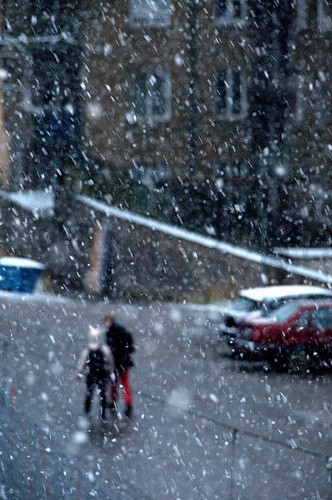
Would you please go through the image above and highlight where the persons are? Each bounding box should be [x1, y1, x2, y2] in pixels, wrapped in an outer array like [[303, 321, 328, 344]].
[[76, 326, 116, 417], [101, 314, 134, 416]]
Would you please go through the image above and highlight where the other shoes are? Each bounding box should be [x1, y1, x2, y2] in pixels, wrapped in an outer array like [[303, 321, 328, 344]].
[[84, 405, 91, 411], [124, 403, 133, 415], [107, 401, 115, 409], [101, 410, 107, 418]]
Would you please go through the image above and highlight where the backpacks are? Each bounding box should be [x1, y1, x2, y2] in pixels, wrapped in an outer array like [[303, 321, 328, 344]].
[[86, 343, 104, 383]]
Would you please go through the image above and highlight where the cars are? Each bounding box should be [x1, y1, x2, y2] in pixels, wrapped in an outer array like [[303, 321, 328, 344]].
[[238, 298, 332, 374]]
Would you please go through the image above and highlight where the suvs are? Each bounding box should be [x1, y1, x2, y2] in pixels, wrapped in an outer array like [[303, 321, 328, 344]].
[[220, 283, 331, 354]]
